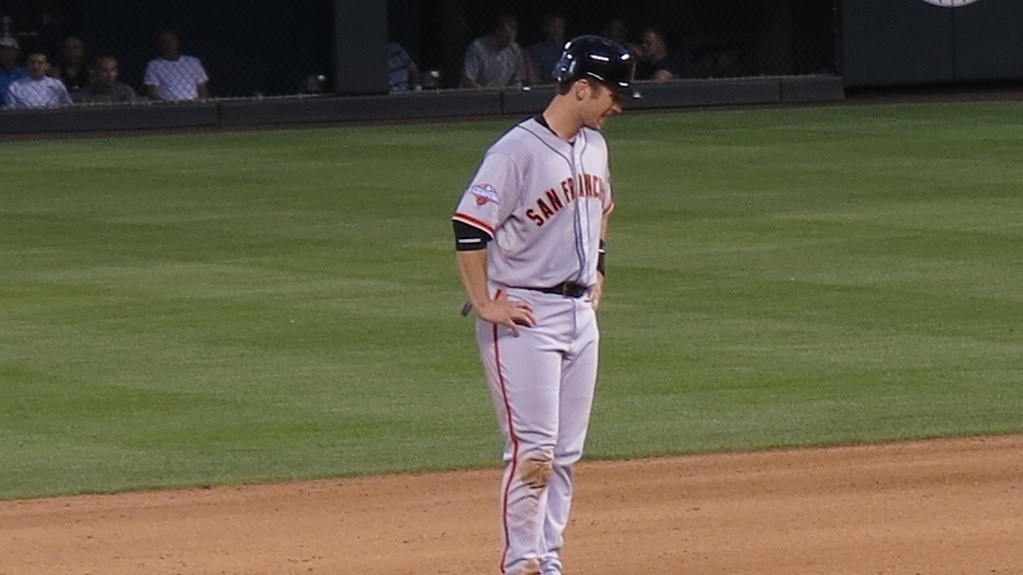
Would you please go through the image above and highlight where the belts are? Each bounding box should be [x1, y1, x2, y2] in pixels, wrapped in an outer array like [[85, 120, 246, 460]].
[[526, 272, 587, 298]]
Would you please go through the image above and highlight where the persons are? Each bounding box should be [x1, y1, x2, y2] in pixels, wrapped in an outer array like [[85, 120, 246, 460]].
[[452, 37, 642, 575], [456, 12, 676, 90], [0, 25, 210, 110]]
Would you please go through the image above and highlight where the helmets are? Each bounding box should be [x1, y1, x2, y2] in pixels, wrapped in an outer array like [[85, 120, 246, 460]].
[[553, 36, 642, 99]]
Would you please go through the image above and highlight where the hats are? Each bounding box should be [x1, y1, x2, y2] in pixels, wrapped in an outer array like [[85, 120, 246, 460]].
[[0, 36, 19, 49]]
